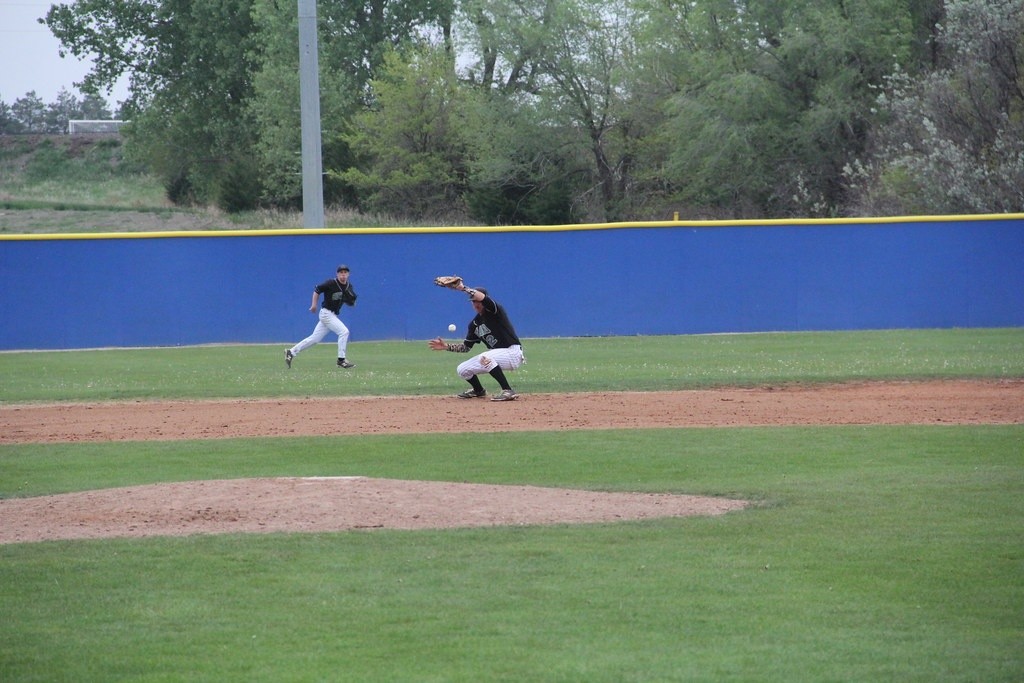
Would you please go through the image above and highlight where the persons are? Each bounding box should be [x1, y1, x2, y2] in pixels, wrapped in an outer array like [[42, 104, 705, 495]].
[[285, 265, 357, 368], [429, 277, 525, 400]]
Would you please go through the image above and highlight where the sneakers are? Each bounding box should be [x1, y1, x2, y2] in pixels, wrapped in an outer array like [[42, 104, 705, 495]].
[[283, 348, 294, 369], [457, 387, 487, 399], [490, 389, 520, 401], [337, 359, 356, 369]]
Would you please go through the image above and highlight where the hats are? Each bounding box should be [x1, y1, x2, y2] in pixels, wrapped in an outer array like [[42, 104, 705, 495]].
[[467, 286, 488, 302], [336, 265, 350, 273]]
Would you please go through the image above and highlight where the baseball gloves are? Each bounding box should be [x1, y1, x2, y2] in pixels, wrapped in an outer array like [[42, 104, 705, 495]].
[[345, 289, 359, 306], [433, 273, 463, 290]]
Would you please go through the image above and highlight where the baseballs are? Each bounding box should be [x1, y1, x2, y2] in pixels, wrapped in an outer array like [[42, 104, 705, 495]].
[[448, 324, 456, 332]]
